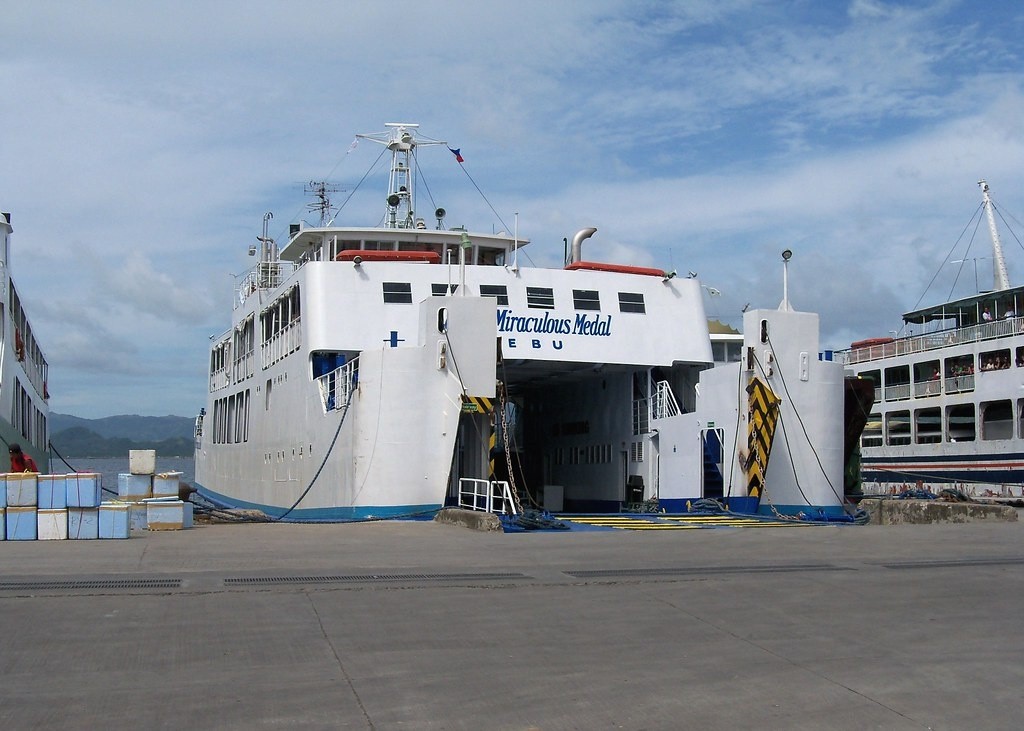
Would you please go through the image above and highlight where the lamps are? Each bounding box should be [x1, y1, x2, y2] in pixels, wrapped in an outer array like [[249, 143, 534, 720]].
[[687, 268, 698, 279], [353, 256, 362, 268], [208, 334, 214, 342], [782, 249, 793, 260], [461, 232, 473, 250], [387, 194, 400, 207]]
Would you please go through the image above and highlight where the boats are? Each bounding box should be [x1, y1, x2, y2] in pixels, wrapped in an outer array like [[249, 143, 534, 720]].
[[190, 122, 876, 533], [709, 180, 1024, 504], [0, 212, 51, 478]]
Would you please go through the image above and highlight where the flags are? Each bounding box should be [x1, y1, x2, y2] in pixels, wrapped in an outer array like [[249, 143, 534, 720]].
[[456, 154, 464, 163], [449, 147, 460, 155]]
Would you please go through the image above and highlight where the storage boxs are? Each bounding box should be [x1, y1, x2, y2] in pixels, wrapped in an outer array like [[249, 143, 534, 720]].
[[38, 509, 68, 540], [152, 475, 178, 498], [37, 475, 66, 508], [102, 502, 147, 529], [66, 473, 103, 508], [118, 474, 151, 502], [6, 506, 37, 540], [147, 499, 183, 531], [129, 449, 155, 474], [0, 508, 6, 541], [99, 505, 132, 539], [69, 507, 99, 539], [0, 471, 7, 508], [7, 472, 42, 506]]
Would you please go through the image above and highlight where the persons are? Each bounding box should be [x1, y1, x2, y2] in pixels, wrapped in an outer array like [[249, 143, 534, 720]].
[[7, 443, 39, 472], [983, 307, 993, 320], [950, 354, 1024, 377], [932, 367, 940, 379], [1000, 306, 1014, 319]]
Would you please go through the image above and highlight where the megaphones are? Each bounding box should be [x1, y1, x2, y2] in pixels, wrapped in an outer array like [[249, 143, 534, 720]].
[[436, 208, 446, 219]]
